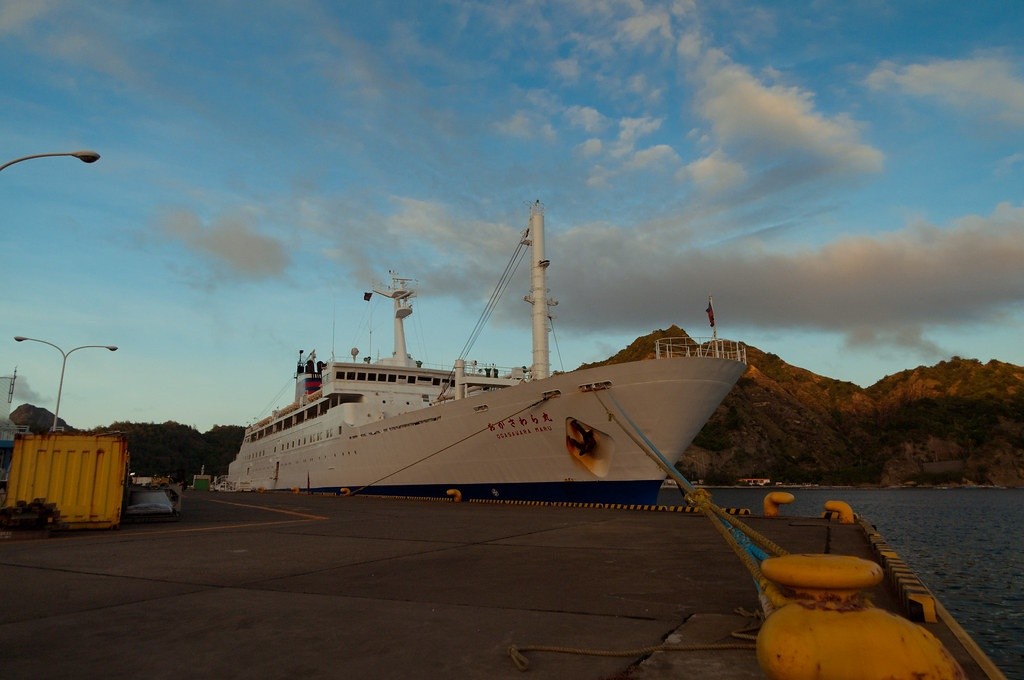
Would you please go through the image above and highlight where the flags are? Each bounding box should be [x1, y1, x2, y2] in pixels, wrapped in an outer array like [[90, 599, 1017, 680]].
[[706, 302, 715, 328], [364, 292, 373, 301]]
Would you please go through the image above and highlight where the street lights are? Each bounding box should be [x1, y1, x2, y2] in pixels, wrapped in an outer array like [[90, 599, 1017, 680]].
[[15, 336, 119, 433]]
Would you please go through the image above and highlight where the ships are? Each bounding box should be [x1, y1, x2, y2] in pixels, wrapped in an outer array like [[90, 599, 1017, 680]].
[[210, 198, 750, 511]]
